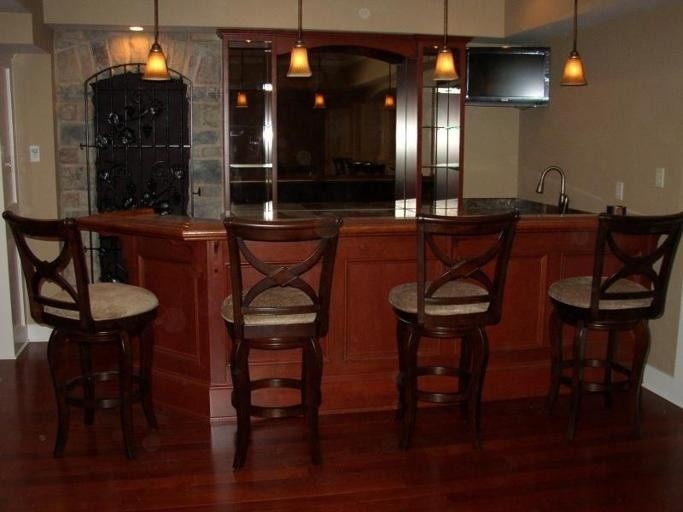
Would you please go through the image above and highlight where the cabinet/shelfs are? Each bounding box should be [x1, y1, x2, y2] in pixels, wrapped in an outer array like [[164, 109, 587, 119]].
[[216, 27, 473, 219]]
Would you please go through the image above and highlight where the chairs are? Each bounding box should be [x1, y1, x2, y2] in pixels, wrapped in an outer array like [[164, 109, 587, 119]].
[[3, 210, 160, 460], [213, 217, 345, 469]]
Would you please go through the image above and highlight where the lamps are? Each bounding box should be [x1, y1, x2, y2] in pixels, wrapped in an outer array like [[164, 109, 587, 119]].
[[233, 48, 395, 112], [285, 1, 316, 81], [430, 0, 457, 84], [561, 1, 588, 86], [140, 1, 171, 83]]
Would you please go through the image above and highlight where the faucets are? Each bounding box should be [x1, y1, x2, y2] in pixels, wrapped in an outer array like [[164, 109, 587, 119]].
[[536, 166, 566, 213]]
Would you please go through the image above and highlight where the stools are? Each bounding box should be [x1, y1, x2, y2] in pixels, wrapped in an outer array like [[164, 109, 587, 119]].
[[547, 211, 683, 441], [386, 211, 520, 450]]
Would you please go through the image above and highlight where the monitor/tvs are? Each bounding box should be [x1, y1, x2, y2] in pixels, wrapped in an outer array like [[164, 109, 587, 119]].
[[465, 46, 552, 106]]
